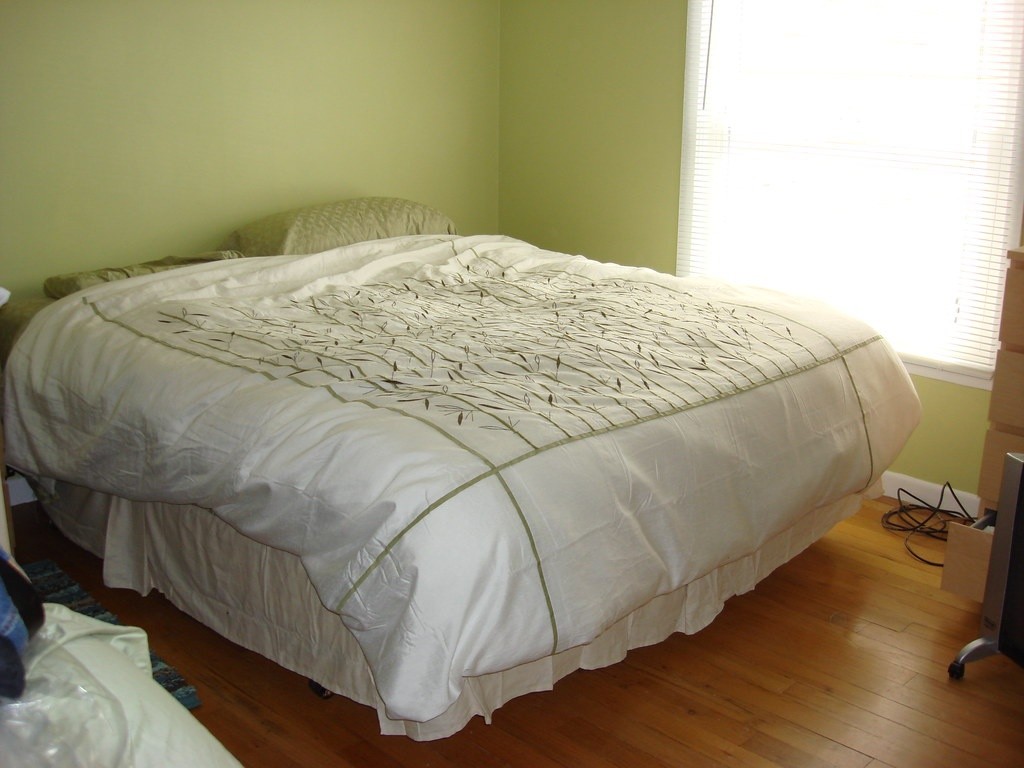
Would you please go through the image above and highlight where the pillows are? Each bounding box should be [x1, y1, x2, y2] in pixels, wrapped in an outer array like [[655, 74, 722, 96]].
[[217, 196, 459, 258]]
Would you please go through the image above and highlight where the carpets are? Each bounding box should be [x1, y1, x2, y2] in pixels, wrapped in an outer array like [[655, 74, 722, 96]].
[[24, 553, 202, 711]]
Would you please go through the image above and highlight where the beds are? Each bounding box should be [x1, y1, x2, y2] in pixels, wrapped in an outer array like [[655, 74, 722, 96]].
[[0, 231, 922, 742]]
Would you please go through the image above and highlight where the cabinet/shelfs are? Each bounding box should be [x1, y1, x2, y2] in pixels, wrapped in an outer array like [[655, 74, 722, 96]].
[[941, 245, 1024, 605]]
